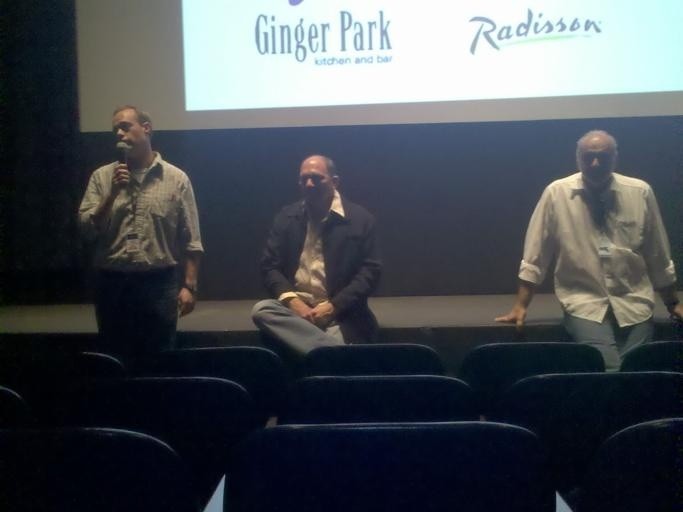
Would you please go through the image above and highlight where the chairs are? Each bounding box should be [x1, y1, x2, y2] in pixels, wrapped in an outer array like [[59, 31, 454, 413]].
[[0, 341, 683, 512]]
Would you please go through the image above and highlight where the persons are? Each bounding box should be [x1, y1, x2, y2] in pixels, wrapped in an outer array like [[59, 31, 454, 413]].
[[74, 105, 204, 377], [248, 155, 385, 362], [490, 129, 683, 373]]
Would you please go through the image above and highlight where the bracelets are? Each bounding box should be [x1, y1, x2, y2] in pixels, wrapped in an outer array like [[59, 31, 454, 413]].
[[181, 281, 200, 295]]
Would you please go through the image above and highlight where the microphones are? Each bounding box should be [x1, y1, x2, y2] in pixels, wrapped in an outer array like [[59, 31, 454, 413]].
[[114, 138, 130, 173]]
